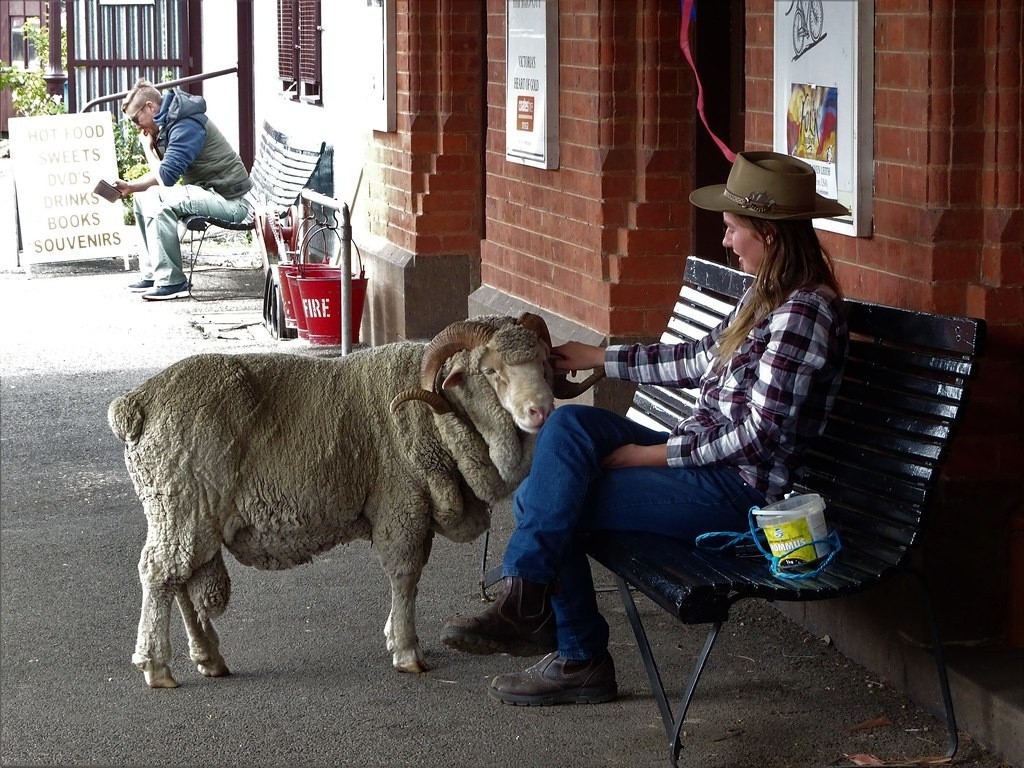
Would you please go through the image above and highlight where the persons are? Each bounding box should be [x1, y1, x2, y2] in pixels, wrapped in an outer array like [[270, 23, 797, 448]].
[[111, 80, 252, 300], [441, 151, 853, 709]]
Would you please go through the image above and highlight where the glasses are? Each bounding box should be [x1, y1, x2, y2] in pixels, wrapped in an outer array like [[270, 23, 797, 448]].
[[130, 103, 146, 125]]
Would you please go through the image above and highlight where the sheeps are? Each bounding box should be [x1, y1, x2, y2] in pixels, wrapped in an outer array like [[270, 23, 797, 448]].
[[106, 312, 607, 690]]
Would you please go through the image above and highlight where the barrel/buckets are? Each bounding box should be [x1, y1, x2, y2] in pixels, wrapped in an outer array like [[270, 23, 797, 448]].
[[277, 217, 369, 344], [756, 494, 832, 573]]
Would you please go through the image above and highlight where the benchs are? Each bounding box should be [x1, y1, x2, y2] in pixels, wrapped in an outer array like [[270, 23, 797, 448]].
[[175, 119, 328, 298], [479, 252, 984, 767]]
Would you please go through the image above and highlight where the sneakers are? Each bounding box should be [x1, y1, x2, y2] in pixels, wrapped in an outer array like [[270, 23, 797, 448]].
[[142, 279, 190, 299], [127, 279, 154, 291]]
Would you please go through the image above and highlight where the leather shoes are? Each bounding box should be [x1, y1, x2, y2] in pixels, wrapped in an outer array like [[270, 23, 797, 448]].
[[488, 650, 617, 706], [440, 577, 560, 656]]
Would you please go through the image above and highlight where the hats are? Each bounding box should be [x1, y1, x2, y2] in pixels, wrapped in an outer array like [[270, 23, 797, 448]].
[[688, 151, 848, 220]]
